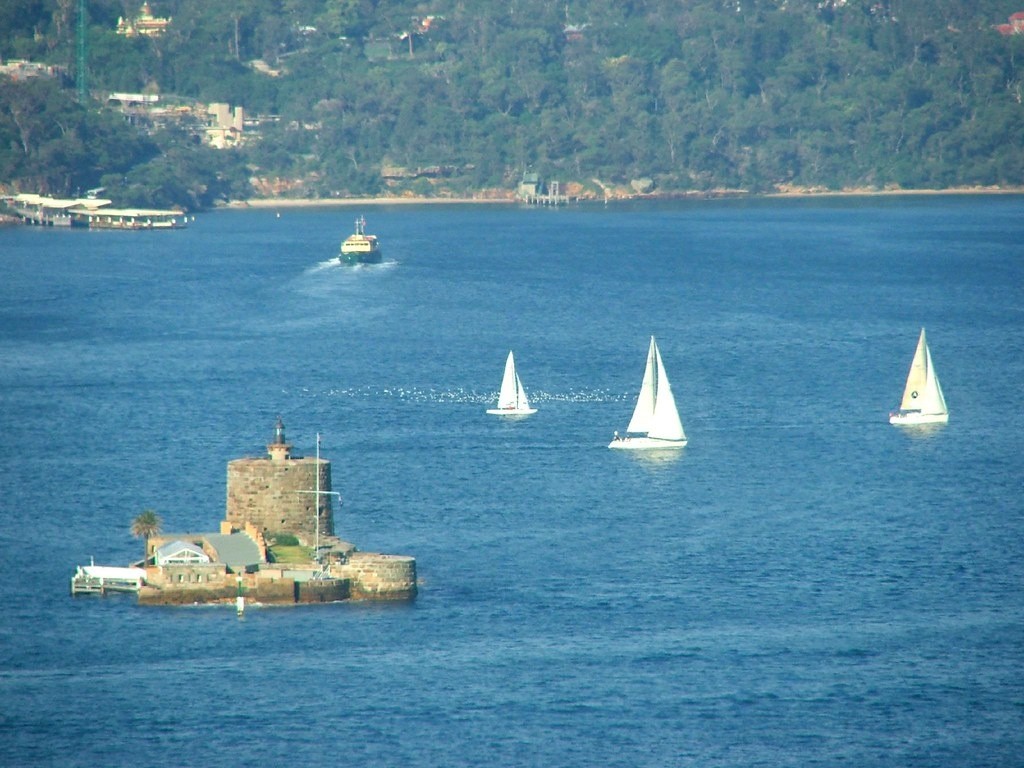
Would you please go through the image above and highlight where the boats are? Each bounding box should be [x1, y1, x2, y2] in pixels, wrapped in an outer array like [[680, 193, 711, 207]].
[[339, 215, 380, 265]]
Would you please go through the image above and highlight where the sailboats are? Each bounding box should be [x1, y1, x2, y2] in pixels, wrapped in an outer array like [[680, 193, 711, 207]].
[[483, 350, 539, 416], [888, 325, 952, 424], [605, 334, 691, 448]]
